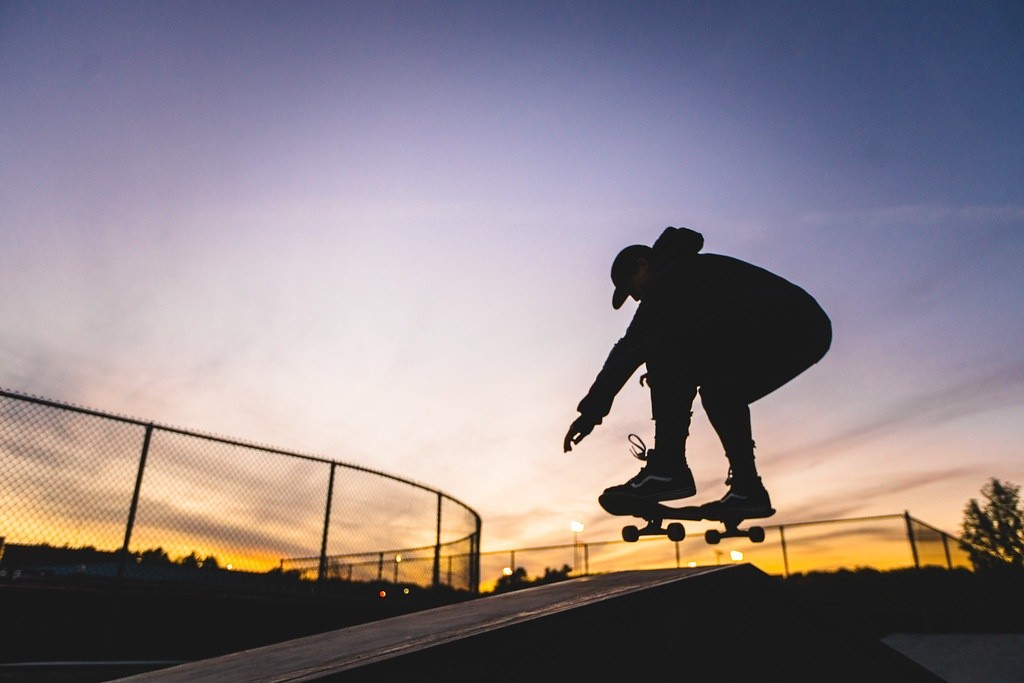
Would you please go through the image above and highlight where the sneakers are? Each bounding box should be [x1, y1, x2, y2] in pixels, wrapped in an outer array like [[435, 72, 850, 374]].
[[604, 434, 696, 501], [701, 475, 774, 519]]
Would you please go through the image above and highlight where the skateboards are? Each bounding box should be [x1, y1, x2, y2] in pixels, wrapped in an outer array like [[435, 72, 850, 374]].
[[598, 488, 776, 544]]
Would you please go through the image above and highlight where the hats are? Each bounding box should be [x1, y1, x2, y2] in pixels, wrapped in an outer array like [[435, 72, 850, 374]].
[[611, 245, 650, 310]]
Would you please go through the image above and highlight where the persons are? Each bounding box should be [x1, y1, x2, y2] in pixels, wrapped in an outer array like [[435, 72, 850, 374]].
[[563, 228, 833, 518]]
